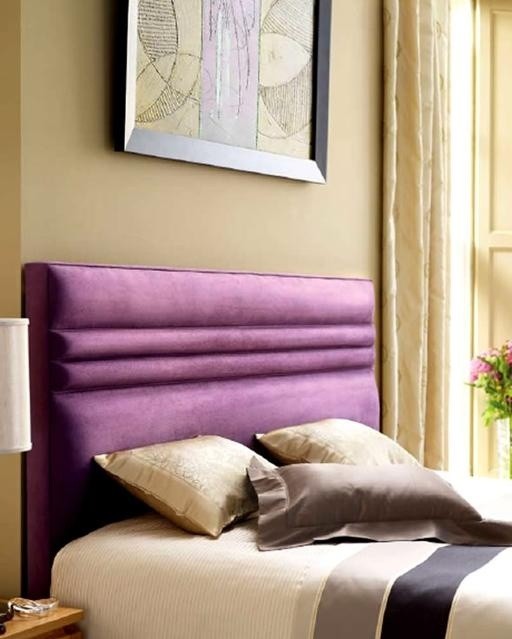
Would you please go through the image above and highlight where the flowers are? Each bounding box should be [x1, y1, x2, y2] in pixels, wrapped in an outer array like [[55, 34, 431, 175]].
[[466, 340, 512, 428]]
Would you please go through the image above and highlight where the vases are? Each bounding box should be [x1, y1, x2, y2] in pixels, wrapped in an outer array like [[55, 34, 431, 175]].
[[494, 421, 512, 482]]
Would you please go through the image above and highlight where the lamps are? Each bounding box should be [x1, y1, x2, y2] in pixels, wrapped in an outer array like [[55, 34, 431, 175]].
[[0, 317, 33, 455]]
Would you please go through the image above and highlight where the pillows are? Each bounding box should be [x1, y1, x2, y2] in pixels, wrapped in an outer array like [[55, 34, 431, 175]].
[[92, 436, 277, 541], [245, 455, 512, 553], [255, 416, 419, 467]]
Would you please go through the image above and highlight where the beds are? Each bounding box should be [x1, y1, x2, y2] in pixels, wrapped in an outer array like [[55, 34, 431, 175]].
[[22, 259, 512, 639]]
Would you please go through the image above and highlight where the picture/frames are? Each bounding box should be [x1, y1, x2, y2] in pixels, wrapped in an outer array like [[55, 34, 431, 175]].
[[110, 0, 332, 186]]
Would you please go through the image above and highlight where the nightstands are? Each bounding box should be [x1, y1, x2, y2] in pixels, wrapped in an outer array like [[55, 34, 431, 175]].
[[0, 603, 86, 639]]
